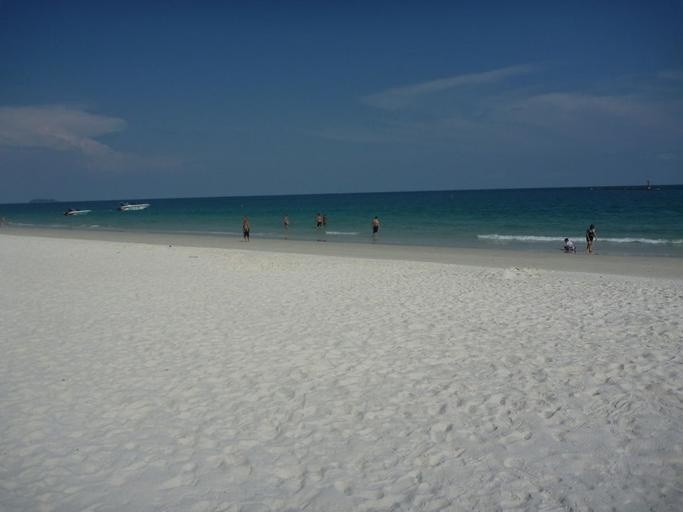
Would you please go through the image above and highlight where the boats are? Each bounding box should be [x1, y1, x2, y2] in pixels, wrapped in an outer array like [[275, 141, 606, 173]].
[[118, 200, 151, 212], [63, 209, 92, 217]]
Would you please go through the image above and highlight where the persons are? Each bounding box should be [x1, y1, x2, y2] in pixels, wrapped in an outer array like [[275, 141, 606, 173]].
[[562, 238, 577, 252], [314, 212, 323, 229], [241, 215, 250, 242], [585, 223, 597, 251], [371, 216, 381, 238], [283, 214, 288, 229]]
